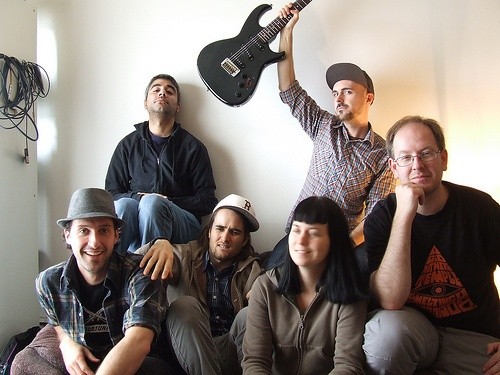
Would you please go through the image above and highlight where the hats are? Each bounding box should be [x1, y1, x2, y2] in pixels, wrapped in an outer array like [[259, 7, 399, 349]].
[[212, 194, 259, 232], [326, 63, 374, 106], [56, 188, 126, 230]]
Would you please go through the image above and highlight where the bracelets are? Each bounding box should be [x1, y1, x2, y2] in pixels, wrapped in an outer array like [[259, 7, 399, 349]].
[[150, 236, 171, 246]]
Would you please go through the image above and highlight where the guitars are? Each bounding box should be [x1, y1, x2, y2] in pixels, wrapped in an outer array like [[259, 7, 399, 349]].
[[196, 0, 313, 108]]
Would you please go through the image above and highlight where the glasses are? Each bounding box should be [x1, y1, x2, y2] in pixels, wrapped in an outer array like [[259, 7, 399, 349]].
[[393, 148, 441, 167]]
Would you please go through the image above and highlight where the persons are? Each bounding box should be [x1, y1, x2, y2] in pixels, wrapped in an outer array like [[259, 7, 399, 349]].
[[34, 188, 186, 375], [241, 195, 368, 375], [362, 115, 500, 375], [105, 74, 220, 253], [133, 194, 268, 375], [265, 3, 397, 280]]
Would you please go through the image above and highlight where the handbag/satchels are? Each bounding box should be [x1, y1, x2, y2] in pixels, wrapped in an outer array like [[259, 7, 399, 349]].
[[0, 326, 42, 375]]
